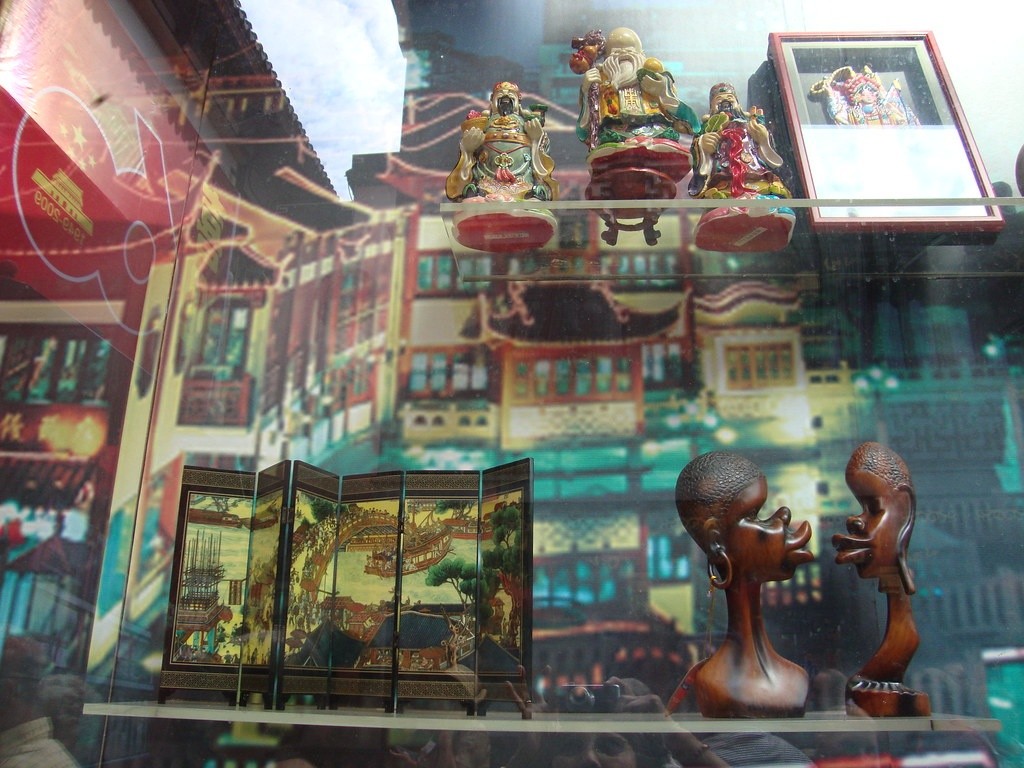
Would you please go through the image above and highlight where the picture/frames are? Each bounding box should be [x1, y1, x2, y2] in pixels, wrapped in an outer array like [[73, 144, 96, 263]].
[[766, 30, 1006, 238]]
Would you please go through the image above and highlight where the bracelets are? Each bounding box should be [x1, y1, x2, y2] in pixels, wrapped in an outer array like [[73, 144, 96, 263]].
[[683, 743, 710, 768]]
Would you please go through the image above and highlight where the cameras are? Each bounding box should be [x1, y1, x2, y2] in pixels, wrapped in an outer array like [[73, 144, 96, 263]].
[[561, 684, 621, 713]]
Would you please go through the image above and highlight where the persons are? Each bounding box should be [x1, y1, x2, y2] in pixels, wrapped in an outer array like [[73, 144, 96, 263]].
[[445, 82, 559, 201], [0, 635, 103, 768], [687, 83, 792, 199], [276, 598, 1024, 768], [810, 66, 922, 125], [569, 28, 702, 152], [831, 440, 931, 719], [675, 449, 814, 717]]
